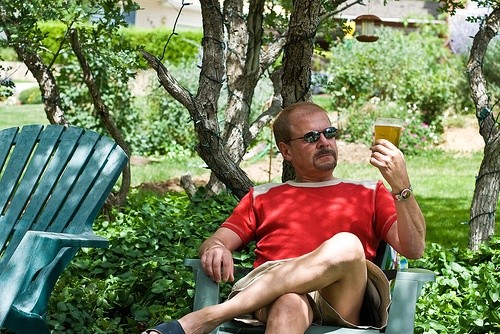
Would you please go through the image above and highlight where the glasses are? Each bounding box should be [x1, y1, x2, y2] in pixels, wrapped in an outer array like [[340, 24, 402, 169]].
[[284, 126, 340, 143]]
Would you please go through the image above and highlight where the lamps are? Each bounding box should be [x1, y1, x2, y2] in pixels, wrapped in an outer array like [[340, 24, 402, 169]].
[[354, 0, 382, 41]]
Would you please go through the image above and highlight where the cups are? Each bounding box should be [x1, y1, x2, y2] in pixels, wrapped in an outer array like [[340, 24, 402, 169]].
[[375, 118, 403, 150]]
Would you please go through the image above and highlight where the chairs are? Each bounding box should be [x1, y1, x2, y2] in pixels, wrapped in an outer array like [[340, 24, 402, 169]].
[[0, 123, 128, 333], [183, 241, 435, 334]]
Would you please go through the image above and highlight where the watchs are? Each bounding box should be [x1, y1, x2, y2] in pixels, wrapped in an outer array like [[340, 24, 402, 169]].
[[393, 186, 413, 202]]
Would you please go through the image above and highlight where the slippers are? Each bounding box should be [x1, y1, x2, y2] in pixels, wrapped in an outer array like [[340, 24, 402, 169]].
[[142, 319, 187, 334]]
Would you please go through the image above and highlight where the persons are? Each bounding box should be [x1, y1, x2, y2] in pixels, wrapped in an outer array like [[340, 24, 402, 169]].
[[141, 102, 426, 334]]
